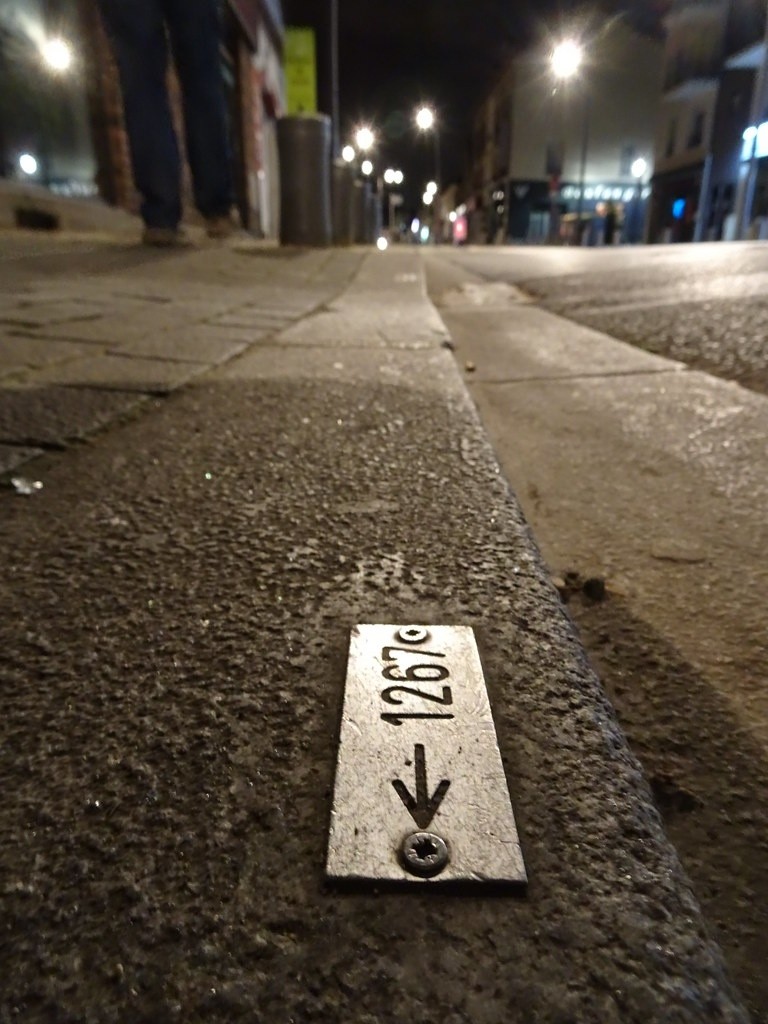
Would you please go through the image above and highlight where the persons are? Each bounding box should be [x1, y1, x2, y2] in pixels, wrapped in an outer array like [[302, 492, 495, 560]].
[[100, 0, 239, 245]]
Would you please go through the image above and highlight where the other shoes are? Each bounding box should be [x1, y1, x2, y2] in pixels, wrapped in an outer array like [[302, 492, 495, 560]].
[[208, 207, 239, 238], [143, 227, 177, 247]]
